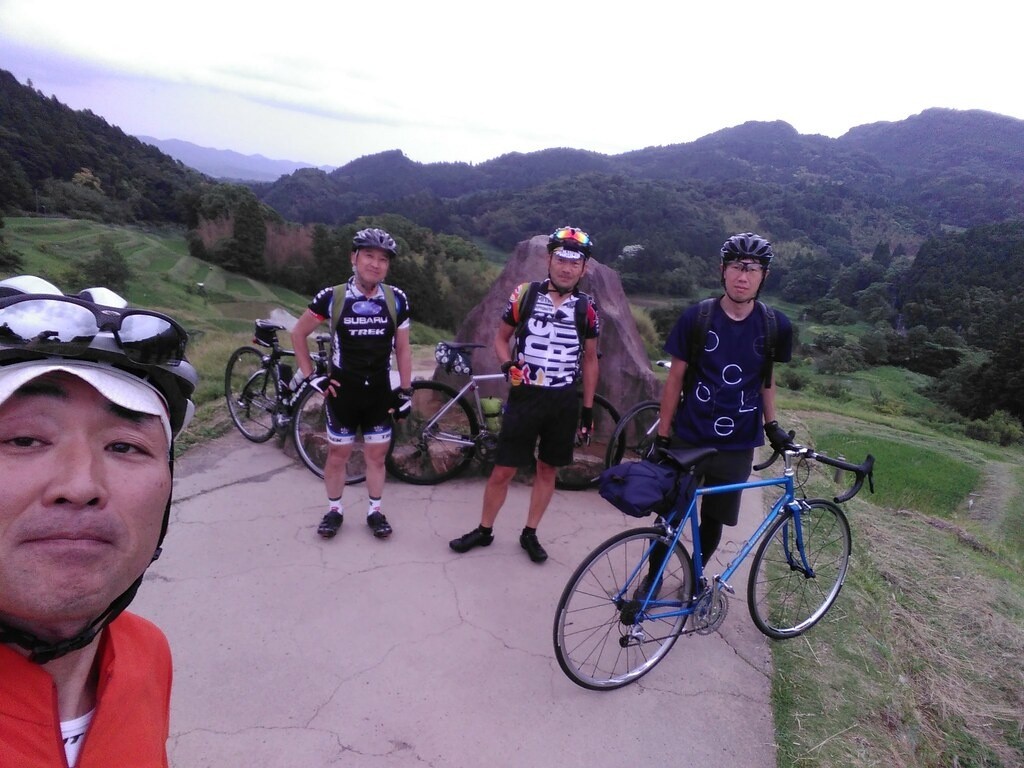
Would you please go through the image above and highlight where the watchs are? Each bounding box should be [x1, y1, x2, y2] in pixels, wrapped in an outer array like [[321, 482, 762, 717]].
[[397, 386, 414, 396]]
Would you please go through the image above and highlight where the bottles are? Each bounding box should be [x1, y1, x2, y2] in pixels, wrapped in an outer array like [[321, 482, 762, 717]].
[[481, 396, 502, 432], [289, 368, 305, 391]]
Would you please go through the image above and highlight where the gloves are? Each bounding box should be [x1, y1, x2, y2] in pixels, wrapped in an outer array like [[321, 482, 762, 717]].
[[645, 434, 668, 462], [764, 420, 795, 452]]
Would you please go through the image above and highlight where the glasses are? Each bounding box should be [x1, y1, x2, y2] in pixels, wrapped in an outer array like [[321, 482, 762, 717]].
[[551, 229, 589, 256], [728, 261, 762, 274], [0, 293, 189, 368]]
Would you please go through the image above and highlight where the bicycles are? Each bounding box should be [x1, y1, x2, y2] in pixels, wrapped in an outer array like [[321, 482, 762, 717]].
[[551, 430, 877, 692], [604, 357, 675, 473], [383, 340, 629, 491], [222, 317, 395, 487]]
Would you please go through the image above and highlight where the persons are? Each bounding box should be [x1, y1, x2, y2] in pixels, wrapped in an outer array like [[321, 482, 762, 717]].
[[450, 226, 599, 562], [634, 232, 794, 609], [0, 276, 198, 768], [291, 228, 412, 537]]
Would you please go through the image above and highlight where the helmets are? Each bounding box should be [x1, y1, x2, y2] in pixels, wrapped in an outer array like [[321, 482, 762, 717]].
[[720, 233, 774, 271], [351, 228, 397, 260], [0, 275, 196, 452], [546, 226, 593, 265]]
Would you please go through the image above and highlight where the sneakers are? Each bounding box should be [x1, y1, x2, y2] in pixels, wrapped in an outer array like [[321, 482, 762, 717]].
[[366, 510, 393, 537], [686, 576, 712, 614], [520, 531, 548, 564], [450, 530, 495, 552], [635, 576, 662, 602], [318, 509, 344, 537]]
